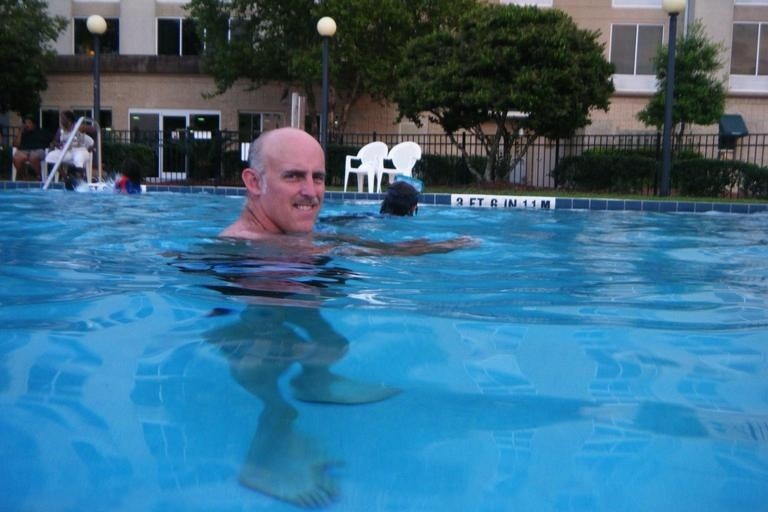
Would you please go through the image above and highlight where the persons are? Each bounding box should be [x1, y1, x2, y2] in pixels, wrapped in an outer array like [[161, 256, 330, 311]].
[[13, 116, 49, 181], [219, 126, 479, 507], [115, 157, 142, 195], [380, 182, 420, 216], [50, 111, 95, 191]]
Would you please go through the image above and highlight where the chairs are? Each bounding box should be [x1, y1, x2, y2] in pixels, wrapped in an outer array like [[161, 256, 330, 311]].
[[11, 133, 94, 184], [342, 141, 421, 194]]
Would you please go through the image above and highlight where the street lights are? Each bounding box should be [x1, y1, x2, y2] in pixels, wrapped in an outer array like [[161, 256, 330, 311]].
[[659, 0, 686, 197], [86, 15, 107, 124], [316, 17, 337, 151]]
